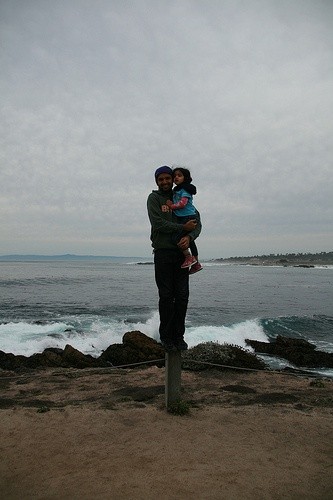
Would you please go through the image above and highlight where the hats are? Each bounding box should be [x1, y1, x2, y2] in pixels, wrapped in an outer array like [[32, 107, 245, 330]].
[[155, 166, 173, 179]]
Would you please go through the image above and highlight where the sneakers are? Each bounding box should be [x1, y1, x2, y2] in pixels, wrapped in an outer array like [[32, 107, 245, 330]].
[[162, 340, 188, 351], [189, 263, 203, 274], [181, 256, 197, 268]]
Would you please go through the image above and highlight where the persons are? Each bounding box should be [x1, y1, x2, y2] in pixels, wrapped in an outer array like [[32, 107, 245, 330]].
[[147, 166, 197, 354], [162, 167, 203, 275]]
